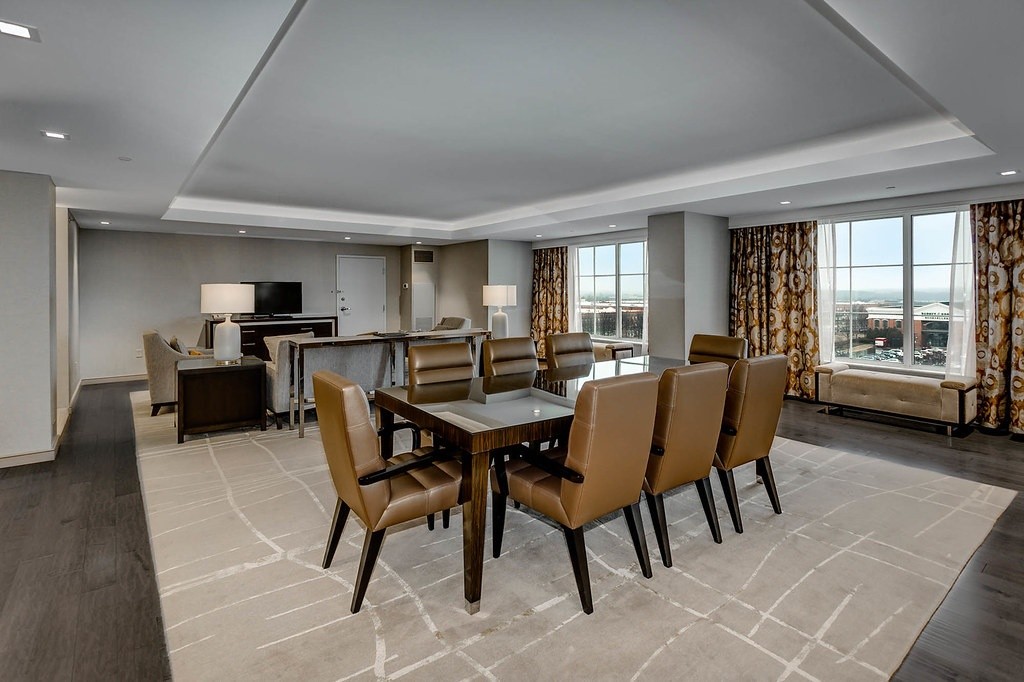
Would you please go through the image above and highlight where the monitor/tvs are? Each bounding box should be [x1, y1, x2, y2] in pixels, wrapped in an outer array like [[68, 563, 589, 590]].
[[240, 282, 302, 318]]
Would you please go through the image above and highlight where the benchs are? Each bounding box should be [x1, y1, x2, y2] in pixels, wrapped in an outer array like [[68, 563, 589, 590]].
[[593, 341, 634, 363], [813, 360, 980, 438]]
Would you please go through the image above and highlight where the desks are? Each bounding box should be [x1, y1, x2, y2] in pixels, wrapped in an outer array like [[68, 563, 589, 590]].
[[374, 354, 706, 615]]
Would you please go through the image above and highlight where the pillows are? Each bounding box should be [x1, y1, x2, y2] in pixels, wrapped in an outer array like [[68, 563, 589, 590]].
[[263, 331, 315, 364]]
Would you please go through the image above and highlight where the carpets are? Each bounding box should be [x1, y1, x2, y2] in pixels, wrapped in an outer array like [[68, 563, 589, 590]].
[[126, 392, 1020, 682]]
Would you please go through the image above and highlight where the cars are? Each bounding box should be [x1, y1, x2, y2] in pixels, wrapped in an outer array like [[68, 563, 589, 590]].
[[855, 345, 943, 364]]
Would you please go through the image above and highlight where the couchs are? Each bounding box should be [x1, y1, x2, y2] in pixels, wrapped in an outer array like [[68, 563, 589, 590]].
[[260, 316, 484, 428], [141, 326, 214, 417]]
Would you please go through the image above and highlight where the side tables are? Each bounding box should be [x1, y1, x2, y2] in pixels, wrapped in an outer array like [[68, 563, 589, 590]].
[[171, 354, 266, 443]]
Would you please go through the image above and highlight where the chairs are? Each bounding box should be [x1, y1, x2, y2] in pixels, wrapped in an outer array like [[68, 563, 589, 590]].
[[408, 331, 596, 529], [710, 353, 788, 535], [491, 371, 661, 615], [641, 360, 731, 567], [312, 368, 464, 615], [689, 333, 749, 367]]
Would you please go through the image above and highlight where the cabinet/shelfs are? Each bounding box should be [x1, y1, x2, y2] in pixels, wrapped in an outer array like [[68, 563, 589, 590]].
[[205, 315, 339, 361]]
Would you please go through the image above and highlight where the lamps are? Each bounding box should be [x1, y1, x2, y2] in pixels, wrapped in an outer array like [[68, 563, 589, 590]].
[[483, 284, 517, 341], [200, 283, 256, 361]]
